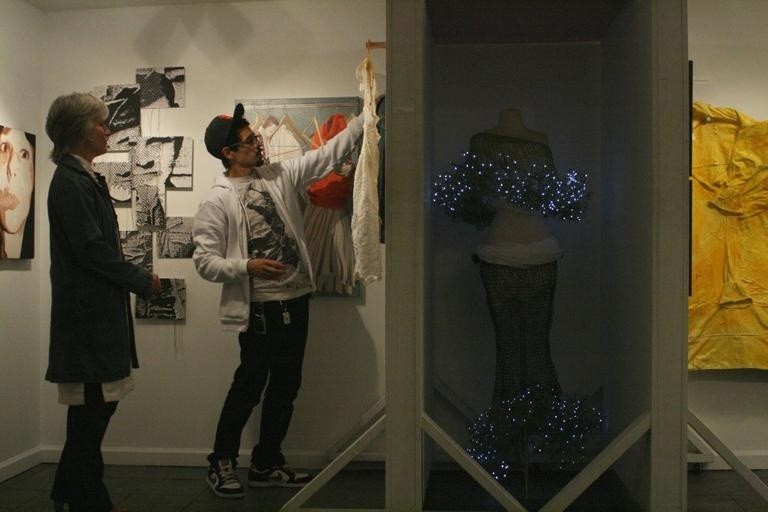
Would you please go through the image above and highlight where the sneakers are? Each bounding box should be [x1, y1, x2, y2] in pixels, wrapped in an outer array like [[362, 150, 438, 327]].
[[204, 455, 246, 499], [246, 462, 312, 489]]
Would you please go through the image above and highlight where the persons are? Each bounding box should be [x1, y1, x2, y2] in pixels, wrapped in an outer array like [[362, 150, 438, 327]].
[[1, 126, 34, 259], [191, 99, 366, 500], [42, 89, 161, 512]]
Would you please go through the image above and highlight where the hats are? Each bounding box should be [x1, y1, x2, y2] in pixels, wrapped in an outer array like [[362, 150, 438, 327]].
[[204, 101, 244, 159]]
